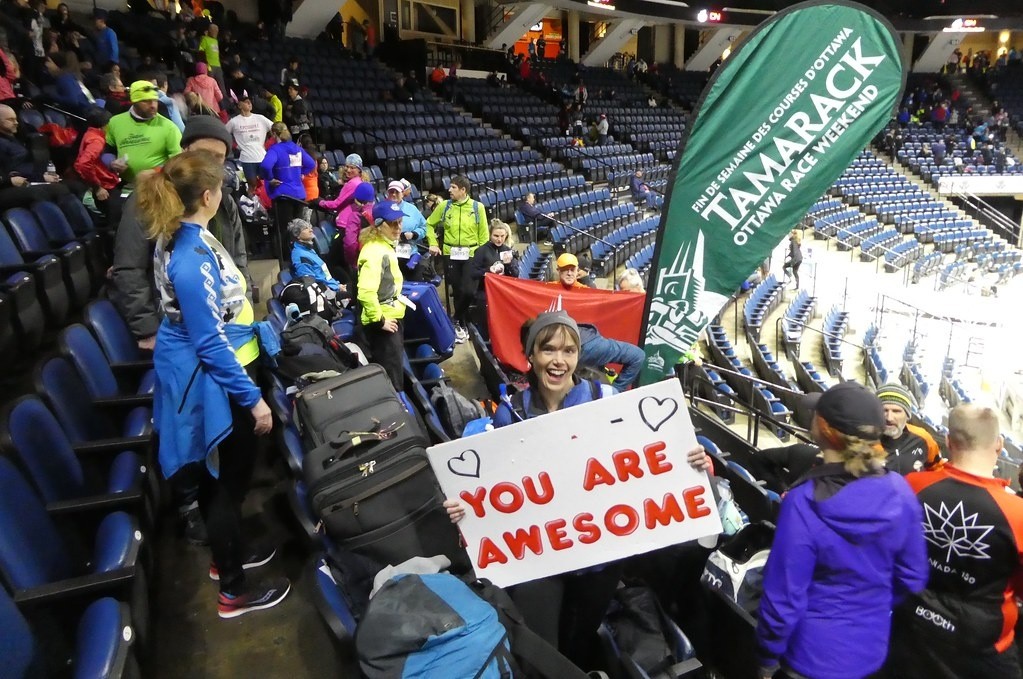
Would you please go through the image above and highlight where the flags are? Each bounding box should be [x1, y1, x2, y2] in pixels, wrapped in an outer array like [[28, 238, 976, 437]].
[[631, 0, 905, 389]]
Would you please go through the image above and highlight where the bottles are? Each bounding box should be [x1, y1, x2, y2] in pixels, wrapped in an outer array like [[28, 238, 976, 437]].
[[698, 534, 719, 548], [47, 160, 56, 178], [406, 252, 420, 270], [589, 271, 596, 283]]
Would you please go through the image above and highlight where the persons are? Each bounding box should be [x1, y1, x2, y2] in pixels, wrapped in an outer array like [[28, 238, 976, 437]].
[[136, 150, 291, 619], [876, 383, 948, 474], [631, 170, 658, 212], [328, 12, 465, 107], [0, 0, 319, 235], [756, 381, 929, 679], [113, 116, 253, 546], [443, 312, 710, 671], [490, 35, 722, 146], [761, 230, 803, 290], [877, 46, 1023, 175], [888, 401, 1023, 679], [288, 152, 645, 394], [736, 444, 824, 497]]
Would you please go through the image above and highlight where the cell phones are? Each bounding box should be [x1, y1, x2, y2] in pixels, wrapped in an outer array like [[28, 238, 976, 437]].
[[120, 153, 128, 164]]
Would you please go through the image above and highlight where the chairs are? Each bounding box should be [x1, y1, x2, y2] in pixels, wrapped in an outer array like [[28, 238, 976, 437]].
[[0, 35, 1023, 679]]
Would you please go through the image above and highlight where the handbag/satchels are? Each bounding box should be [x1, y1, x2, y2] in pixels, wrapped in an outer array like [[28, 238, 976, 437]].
[[355, 572, 518, 679]]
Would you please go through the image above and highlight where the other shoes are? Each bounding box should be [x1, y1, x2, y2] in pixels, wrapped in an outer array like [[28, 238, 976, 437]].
[[183, 508, 209, 547], [454, 325, 470, 343]]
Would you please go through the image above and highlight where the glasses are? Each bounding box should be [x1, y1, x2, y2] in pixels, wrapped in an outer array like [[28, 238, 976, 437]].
[[140, 86, 158, 92]]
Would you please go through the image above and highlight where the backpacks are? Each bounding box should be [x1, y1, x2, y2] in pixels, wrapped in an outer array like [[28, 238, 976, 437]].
[[279, 312, 364, 385], [278, 274, 342, 326]]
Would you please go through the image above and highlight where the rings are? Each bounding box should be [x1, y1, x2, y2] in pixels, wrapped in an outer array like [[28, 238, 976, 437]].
[[263, 422, 267, 424]]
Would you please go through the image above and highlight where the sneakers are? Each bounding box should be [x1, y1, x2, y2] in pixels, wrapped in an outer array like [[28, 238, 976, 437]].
[[207, 546, 277, 580], [218, 575, 292, 619]]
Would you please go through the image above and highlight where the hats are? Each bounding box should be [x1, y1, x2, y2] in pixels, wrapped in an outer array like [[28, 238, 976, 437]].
[[371, 200, 410, 222], [129, 80, 159, 104], [355, 182, 375, 201], [525, 311, 579, 356], [346, 153, 363, 173], [802, 381, 887, 439], [872, 383, 913, 420], [196, 61, 207, 74], [388, 180, 404, 192], [558, 253, 579, 267], [180, 115, 231, 157]]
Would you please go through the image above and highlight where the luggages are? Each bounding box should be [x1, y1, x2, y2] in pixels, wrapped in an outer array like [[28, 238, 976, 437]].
[[402, 280, 456, 354], [297, 362, 470, 601]]
